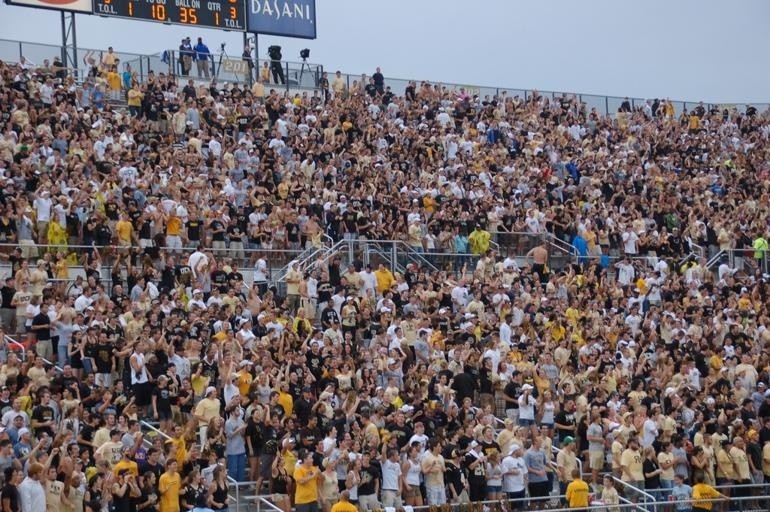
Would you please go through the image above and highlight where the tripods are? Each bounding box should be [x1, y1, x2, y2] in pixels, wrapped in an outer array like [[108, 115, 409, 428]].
[[298, 58, 318, 87], [216, 48, 240, 82]]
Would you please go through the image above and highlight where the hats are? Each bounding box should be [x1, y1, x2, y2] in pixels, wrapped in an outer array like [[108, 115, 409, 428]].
[[0, 253, 769, 480]]
